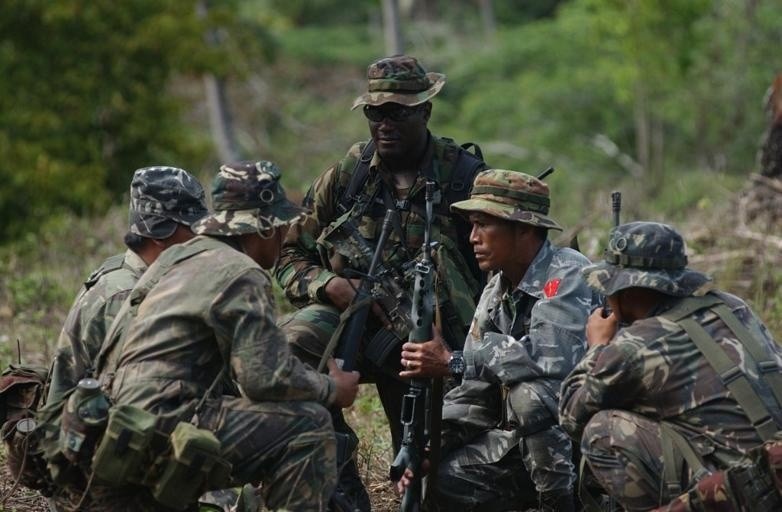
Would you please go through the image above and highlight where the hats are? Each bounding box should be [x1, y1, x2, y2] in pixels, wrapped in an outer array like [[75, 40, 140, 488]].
[[350, 54, 447, 111], [128, 166, 208, 240], [191, 160, 313, 237], [449, 169, 564, 232], [577, 221, 712, 298]]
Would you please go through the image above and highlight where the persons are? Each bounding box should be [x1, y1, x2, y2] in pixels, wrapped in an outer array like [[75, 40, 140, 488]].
[[556, 222, 782, 512], [93, 160, 360, 512], [272, 54, 500, 511], [42, 165, 211, 499], [397, 170, 629, 512]]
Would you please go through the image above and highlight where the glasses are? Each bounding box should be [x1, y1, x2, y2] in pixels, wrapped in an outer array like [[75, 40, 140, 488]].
[[363, 104, 422, 122]]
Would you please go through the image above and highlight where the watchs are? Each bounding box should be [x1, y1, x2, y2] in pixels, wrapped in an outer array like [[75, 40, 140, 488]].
[[448, 350, 467, 381]]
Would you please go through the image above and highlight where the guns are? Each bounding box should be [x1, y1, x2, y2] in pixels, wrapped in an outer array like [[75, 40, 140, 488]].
[[324, 166, 555, 511], [589, 190, 621, 330]]
